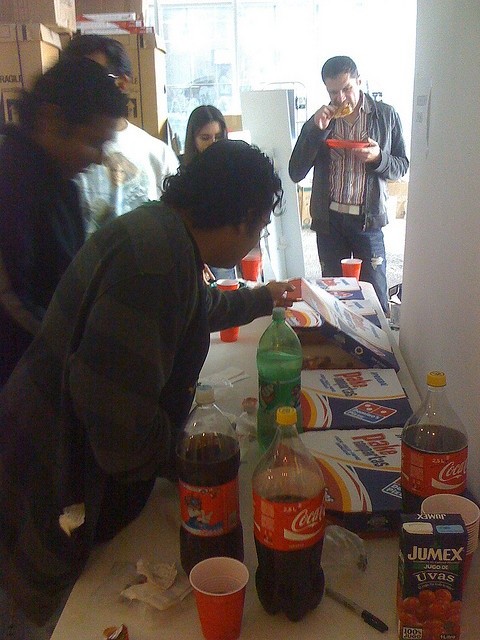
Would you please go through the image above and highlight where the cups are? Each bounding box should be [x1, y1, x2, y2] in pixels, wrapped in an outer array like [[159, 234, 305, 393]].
[[188, 557, 250, 640], [220, 327, 239, 342], [241, 257, 259, 281], [341, 259, 362, 280], [421, 494, 480, 594], [217, 278, 239, 290]]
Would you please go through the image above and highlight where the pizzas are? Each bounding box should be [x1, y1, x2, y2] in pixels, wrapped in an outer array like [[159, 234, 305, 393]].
[[332, 104, 354, 119], [302, 356, 368, 369]]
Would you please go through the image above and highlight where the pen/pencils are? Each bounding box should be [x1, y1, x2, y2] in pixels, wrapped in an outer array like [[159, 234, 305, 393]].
[[325, 587, 388, 632]]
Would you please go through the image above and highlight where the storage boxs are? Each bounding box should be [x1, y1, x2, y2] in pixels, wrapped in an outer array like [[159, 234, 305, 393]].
[[0, 24, 61, 126], [297, 184, 312, 230], [76, 1, 145, 27], [0, 0, 76, 33], [382, 178, 408, 219], [61, 34, 168, 145]]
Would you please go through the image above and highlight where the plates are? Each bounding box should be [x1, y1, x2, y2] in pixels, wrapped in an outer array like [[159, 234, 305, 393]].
[[326, 138, 370, 149]]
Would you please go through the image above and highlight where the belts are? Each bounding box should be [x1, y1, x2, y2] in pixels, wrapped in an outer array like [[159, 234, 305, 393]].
[[329, 201, 360, 215]]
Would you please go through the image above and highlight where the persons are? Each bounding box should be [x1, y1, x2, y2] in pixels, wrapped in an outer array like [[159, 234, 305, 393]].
[[55, 31, 180, 240], [287, 54, 409, 314], [1, 52, 131, 384], [177, 103, 237, 287], [0, 138, 297, 640]]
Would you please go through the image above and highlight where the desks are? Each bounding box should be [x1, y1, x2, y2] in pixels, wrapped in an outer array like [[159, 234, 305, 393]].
[[51, 281, 480, 639]]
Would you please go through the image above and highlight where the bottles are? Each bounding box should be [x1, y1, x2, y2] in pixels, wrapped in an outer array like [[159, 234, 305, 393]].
[[174, 384, 245, 579], [251, 406, 325, 622], [402, 370, 469, 513], [256, 307, 303, 448]]
